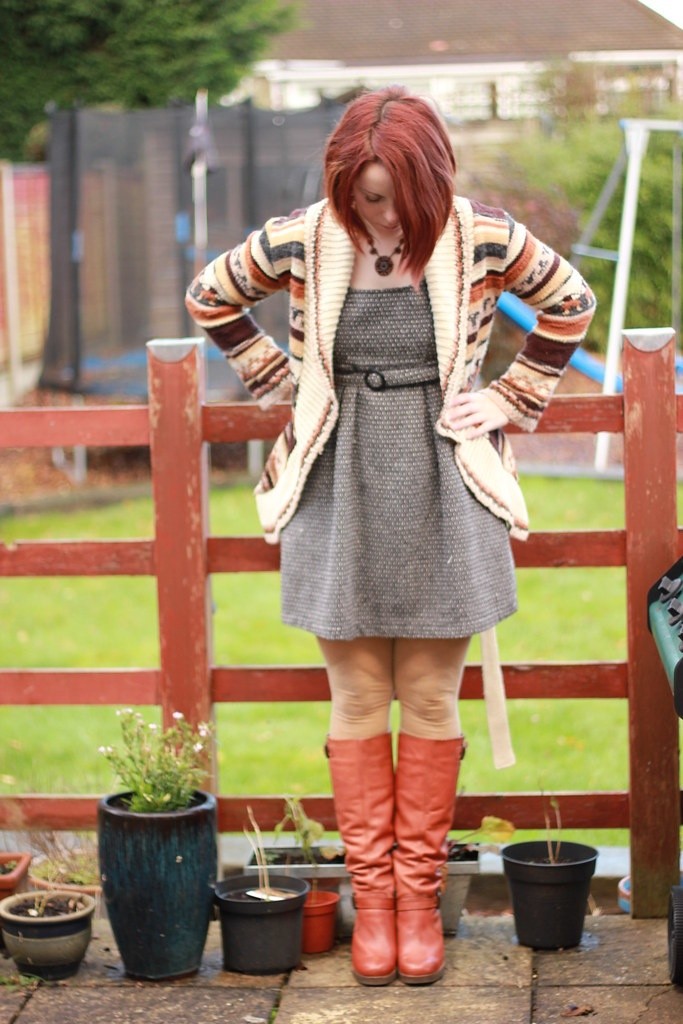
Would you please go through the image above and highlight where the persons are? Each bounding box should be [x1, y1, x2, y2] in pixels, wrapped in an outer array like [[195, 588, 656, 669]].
[[180, 85, 600, 986]]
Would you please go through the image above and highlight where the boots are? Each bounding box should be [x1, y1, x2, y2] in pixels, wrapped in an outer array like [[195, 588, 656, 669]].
[[392, 732, 467, 985], [323, 732, 397, 986]]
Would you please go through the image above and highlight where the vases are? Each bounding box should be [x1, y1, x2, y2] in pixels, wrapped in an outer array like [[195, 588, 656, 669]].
[[0, 890, 96, 982], [0, 851, 32, 901], [302, 890, 340, 952], [96, 786, 219, 981]]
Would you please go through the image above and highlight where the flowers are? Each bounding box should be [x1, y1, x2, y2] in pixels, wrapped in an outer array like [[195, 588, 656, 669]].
[[97, 702, 218, 806]]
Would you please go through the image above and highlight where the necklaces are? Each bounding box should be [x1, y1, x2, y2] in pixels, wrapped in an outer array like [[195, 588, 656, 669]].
[[364, 227, 406, 277]]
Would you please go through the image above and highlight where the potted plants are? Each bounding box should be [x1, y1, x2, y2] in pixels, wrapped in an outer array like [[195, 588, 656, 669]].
[[500, 776, 598, 948], [243, 783, 480, 936], [212, 806, 311, 977]]
